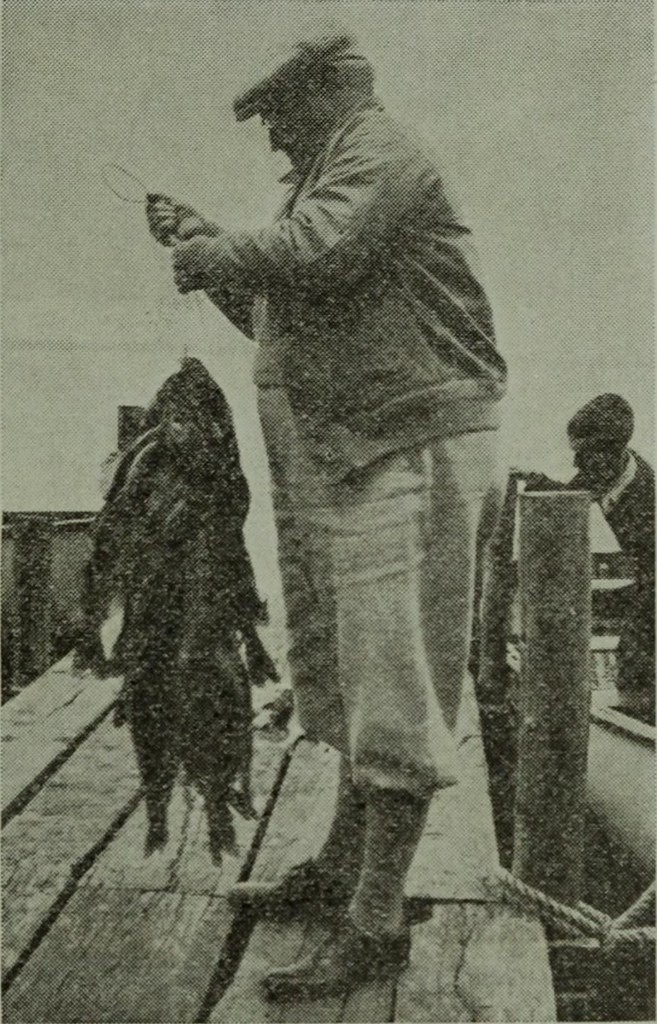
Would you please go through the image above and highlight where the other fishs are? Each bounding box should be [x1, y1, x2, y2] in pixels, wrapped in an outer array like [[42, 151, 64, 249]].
[[63, 359, 278, 863]]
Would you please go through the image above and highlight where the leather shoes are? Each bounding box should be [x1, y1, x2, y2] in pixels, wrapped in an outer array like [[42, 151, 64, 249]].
[[261, 921, 410, 999], [222, 857, 353, 927]]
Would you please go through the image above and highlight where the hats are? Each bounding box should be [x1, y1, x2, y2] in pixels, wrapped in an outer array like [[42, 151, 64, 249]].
[[225, 21, 362, 128], [566, 393, 633, 446]]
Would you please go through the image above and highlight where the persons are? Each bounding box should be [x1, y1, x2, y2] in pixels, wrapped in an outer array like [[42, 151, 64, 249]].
[[508, 394, 657, 726], [143, 28, 508, 1002]]
